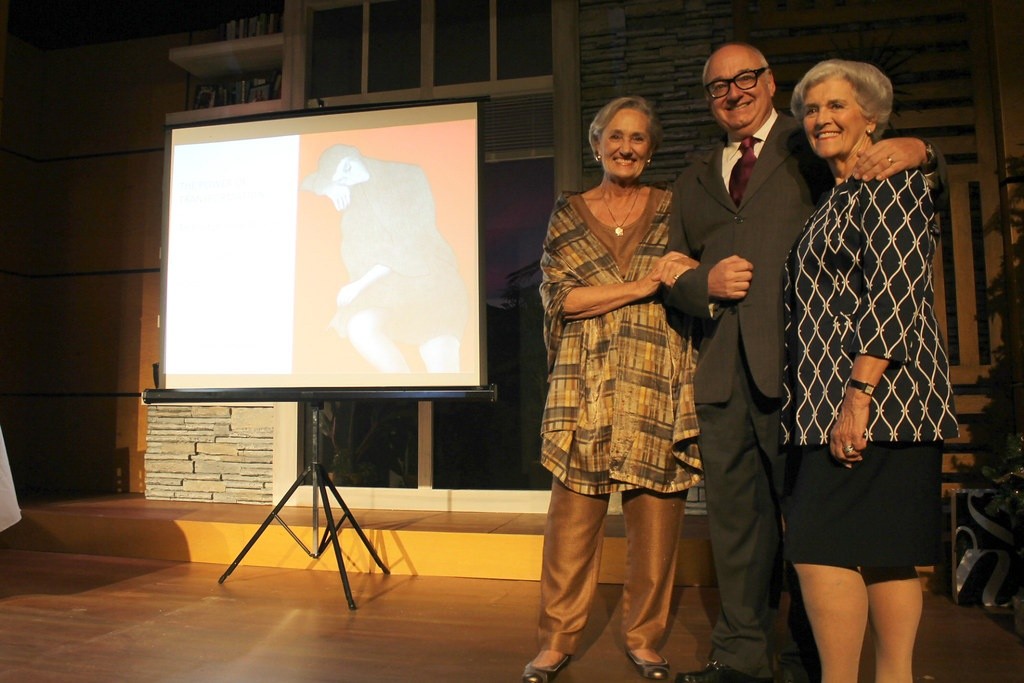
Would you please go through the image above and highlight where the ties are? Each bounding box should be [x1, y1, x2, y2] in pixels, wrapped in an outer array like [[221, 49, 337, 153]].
[[729, 137, 763, 207]]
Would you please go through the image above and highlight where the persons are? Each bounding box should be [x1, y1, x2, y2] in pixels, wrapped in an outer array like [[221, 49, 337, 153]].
[[777, 61, 960, 683], [661, 42, 948, 683], [524, 96, 701, 682]]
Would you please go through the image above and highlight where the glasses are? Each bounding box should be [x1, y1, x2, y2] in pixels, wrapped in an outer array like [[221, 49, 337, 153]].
[[705, 67, 766, 99]]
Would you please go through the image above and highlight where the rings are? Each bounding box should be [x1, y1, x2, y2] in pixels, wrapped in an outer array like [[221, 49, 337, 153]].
[[843, 446, 854, 454], [887, 156, 894, 165], [673, 274, 679, 280]]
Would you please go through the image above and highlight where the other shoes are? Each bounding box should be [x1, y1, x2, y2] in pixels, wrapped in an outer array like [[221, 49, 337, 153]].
[[521, 655, 570, 683], [625, 647, 670, 680]]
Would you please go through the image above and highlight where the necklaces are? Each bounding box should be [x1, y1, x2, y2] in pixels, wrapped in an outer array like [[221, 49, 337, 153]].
[[599, 182, 642, 236]]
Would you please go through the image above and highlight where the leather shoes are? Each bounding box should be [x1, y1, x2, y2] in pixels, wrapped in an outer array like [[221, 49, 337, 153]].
[[675, 659, 775, 683]]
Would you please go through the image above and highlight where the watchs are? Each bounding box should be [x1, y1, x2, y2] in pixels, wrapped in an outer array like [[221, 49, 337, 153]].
[[847, 378, 876, 397]]
